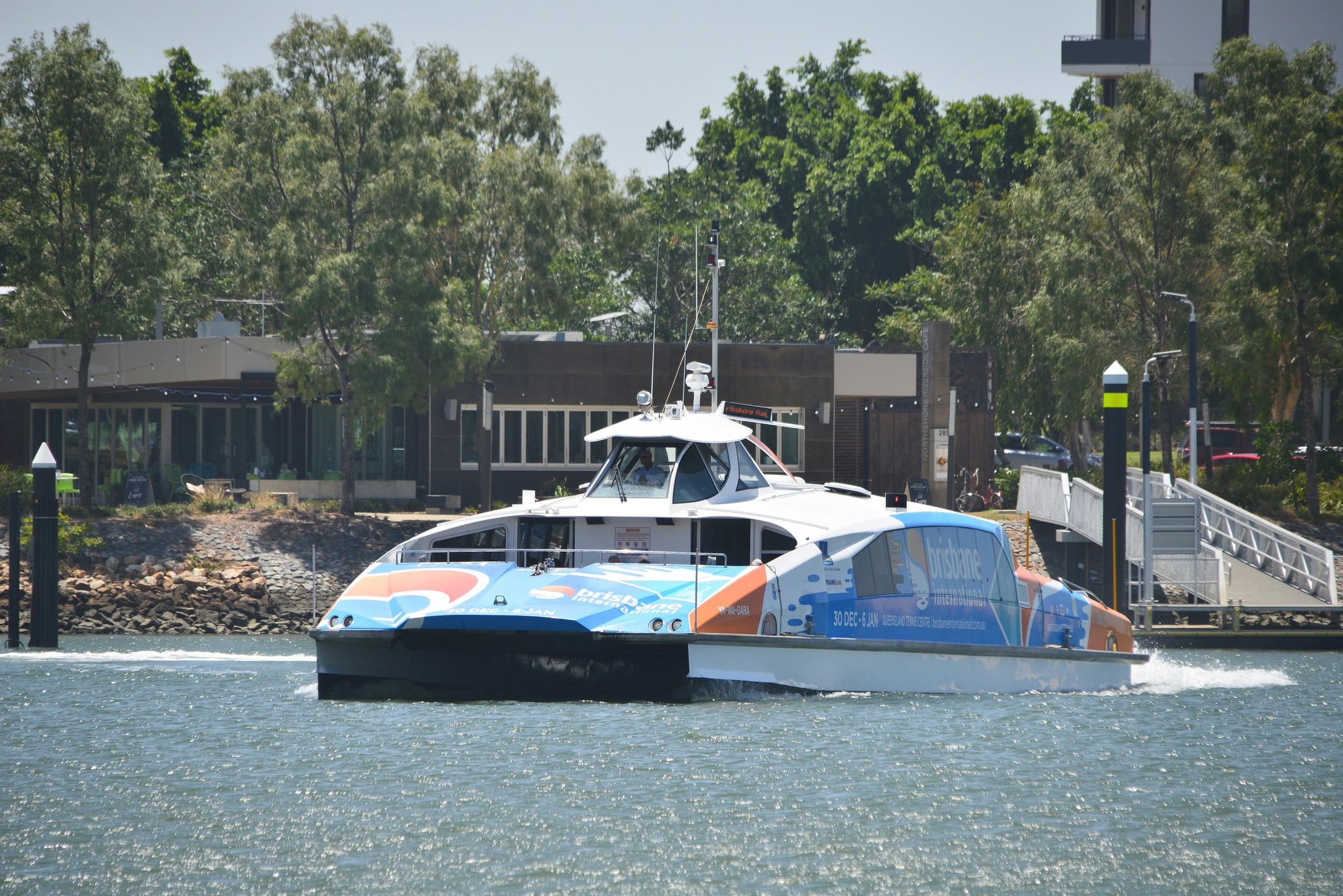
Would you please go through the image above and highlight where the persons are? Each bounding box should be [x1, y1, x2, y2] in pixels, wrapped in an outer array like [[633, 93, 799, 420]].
[[630, 450, 666, 487], [639, 559, 651, 564], [608, 556, 620, 563]]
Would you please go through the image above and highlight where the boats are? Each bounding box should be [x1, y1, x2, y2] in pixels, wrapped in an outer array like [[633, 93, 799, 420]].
[[309, 219, 1150, 703]]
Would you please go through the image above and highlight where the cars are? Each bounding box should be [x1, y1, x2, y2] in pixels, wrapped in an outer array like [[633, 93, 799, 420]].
[[1177, 419, 1343, 478]]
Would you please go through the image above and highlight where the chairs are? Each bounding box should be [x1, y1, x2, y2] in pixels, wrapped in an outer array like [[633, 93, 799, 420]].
[[23, 468, 134, 507], [163, 463, 192, 503], [189, 462, 215, 484], [180, 472, 204, 492]]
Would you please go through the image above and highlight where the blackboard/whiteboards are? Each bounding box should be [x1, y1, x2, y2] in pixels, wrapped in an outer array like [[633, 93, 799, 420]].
[[904, 478, 931, 505], [124, 471, 156, 507]]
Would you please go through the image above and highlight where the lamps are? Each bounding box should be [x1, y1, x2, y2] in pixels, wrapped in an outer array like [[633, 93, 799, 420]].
[[637, 390, 651, 405]]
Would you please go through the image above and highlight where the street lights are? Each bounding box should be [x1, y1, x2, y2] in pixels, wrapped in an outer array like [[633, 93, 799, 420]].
[[1159, 291, 1197, 484], [1142, 350, 1183, 631]]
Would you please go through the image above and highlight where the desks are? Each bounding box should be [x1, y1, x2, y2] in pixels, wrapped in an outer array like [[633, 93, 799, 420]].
[[202, 479, 236, 499]]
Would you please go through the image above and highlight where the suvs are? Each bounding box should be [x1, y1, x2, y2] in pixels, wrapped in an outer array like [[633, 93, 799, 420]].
[[993, 432, 1103, 473]]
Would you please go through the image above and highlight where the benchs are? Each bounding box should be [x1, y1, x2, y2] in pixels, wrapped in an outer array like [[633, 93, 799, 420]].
[[267, 492, 298, 505]]
